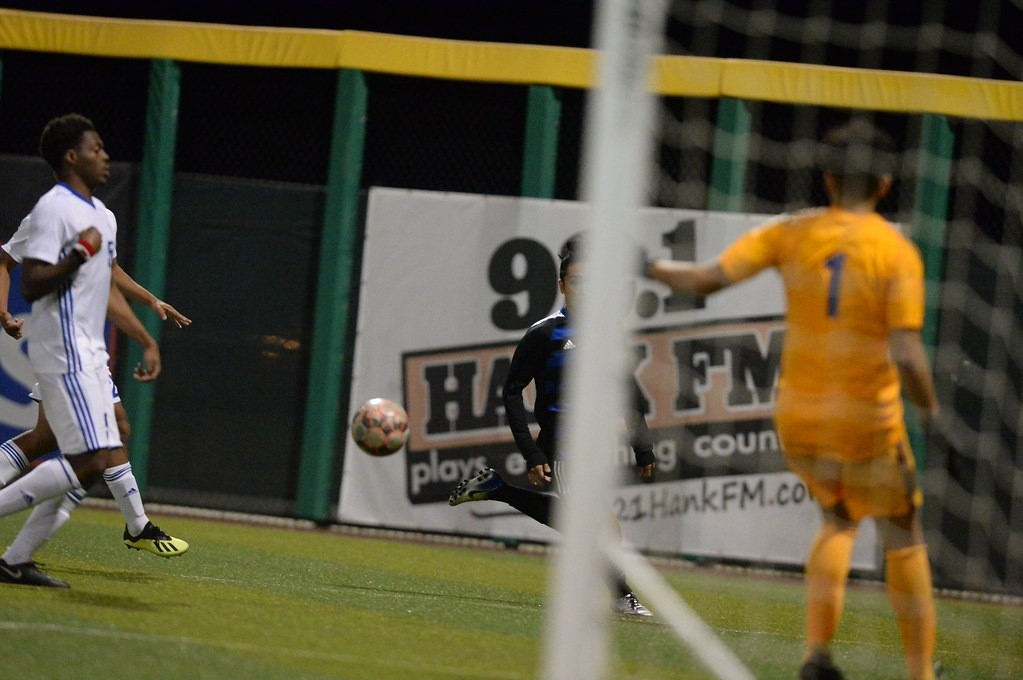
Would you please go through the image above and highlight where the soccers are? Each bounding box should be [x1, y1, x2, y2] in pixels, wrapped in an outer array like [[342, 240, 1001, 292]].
[[354, 398, 410, 455]]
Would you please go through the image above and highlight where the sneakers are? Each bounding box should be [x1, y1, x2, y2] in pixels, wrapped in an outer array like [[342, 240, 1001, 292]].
[[0, 558, 71, 588], [449, 468, 502, 506], [124, 520, 187, 558], [611, 593, 654, 617]]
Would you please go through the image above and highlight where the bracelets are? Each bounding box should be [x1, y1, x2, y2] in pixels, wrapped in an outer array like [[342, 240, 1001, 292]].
[[79, 240, 96, 256], [74, 243, 92, 262], [644, 255, 658, 279]]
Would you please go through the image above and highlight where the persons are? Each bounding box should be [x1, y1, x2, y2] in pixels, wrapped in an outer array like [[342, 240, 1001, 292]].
[[450, 248, 655, 619], [0, 114, 194, 588], [639, 120, 945, 680]]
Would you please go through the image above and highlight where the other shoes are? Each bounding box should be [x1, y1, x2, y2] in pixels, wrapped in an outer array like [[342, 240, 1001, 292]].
[[801, 660, 843, 680]]
